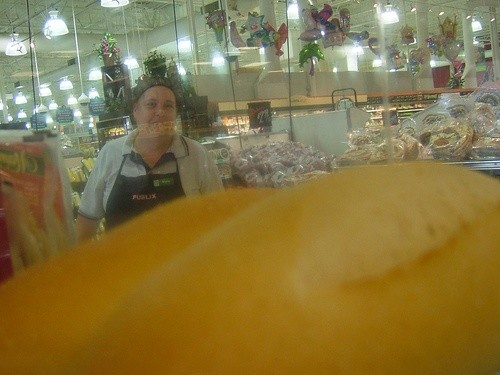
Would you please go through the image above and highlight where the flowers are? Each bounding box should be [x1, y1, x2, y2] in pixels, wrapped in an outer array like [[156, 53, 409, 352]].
[[96, 32, 117, 52]]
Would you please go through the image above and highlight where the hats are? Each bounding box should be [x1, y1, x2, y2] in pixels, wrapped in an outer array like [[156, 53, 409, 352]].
[[134, 76, 172, 104]]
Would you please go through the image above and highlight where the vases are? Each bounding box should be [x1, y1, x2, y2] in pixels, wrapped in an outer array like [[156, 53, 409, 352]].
[[102, 53, 114, 66]]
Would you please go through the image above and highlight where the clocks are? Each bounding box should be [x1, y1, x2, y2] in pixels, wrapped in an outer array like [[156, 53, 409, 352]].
[[335, 97, 355, 110]]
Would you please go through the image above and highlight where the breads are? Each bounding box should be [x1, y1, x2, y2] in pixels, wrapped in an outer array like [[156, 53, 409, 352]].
[[343, 91, 500, 163], [0, 164, 500, 375]]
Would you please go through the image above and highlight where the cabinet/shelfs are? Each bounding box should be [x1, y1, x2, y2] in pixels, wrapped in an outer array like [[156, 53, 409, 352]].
[[366, 108, 396, 127], [397, 109, 424, 123]]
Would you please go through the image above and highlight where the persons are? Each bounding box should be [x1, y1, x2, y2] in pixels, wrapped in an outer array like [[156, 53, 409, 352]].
[[74, 79, 225, 245], [456, 46, 490, 87]]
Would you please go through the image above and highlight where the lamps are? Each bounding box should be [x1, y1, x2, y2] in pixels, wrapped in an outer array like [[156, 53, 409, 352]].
[[381, 5, 398, 24], [6, 33, 27, 56], [101, 0, 129, 7], [44, 11, 69, 36], [0, 57, 139, 128]]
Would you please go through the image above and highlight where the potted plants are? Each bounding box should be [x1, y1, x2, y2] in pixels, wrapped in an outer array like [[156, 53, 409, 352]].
[[143, 50, 167, 78]]
